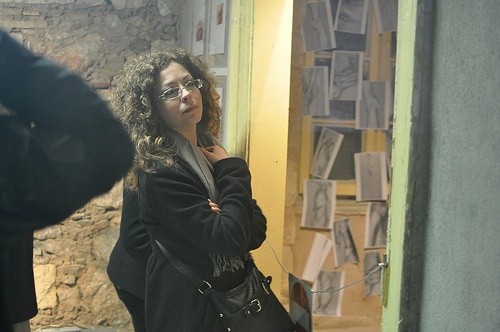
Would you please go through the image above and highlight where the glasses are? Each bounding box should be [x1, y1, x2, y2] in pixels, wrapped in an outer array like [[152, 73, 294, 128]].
[[154, 79, 203, 101]]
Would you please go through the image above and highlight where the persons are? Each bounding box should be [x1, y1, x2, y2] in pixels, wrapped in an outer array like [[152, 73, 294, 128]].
[[106, 47, 298, 332], [0, 27, 136, 332]]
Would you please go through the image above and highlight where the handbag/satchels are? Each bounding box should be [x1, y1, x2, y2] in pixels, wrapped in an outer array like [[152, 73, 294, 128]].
[[202, 260, 294, 332]]
